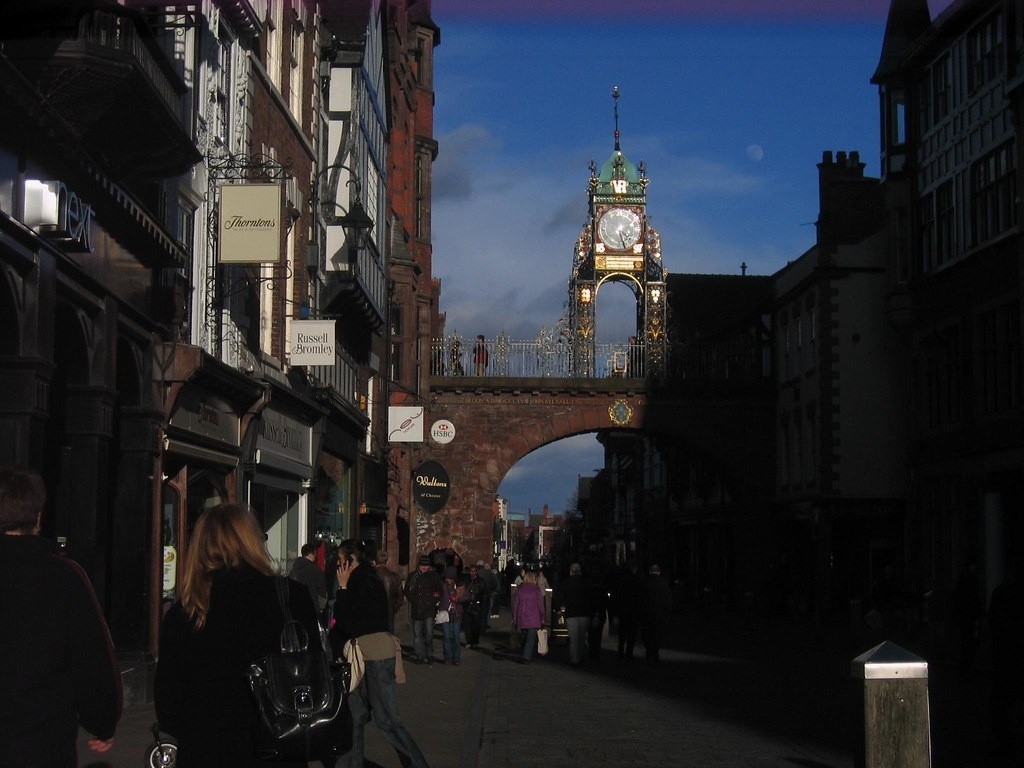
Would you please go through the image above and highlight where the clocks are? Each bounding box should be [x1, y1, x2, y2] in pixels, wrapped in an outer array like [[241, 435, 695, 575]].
[[598, 207, 641, 250]]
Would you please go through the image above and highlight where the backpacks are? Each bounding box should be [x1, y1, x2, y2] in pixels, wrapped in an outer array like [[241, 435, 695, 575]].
[[251, 575, 354, 765]]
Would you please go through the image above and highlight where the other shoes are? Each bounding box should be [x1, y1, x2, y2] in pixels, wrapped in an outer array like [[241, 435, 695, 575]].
[[454, 661, 459, 664], [416, 659, 423, 663], [428, 657, 433, 664], [445, 660, 450, 665]]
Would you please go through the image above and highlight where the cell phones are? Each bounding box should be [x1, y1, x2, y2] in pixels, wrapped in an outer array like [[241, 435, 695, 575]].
[[341, 556, 354, 572]]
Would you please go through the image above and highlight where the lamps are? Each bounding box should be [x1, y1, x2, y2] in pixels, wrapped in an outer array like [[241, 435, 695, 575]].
[[312, 165, 373, 243]]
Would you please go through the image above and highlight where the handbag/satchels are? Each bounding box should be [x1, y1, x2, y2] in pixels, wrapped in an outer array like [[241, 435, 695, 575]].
[[537, 625, 548, 655]]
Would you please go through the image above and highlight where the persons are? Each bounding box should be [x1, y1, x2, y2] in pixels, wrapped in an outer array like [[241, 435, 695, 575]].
[[459, 562, 503, 650], [951, 557, 981, 675], [626, 336, 645, 377], [434, 566, 467, 665], [504, 557, 567, 596], [150, 505, 354, 768], [374, 551, 404, 635], [285, 526, 341, 618], [329, 534, 429, 768], [472, 335, 487, 376], [573, 556, 672, 665], [511, 573, 546, 664], [552, 563, 597, 669], [0, 465, 124, 768], [450, 342, 465, 376], [404, 555, 444, 665]]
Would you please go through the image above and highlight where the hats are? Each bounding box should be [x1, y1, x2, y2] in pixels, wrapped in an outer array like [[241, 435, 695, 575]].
[[418, 556, 431, 566]]
